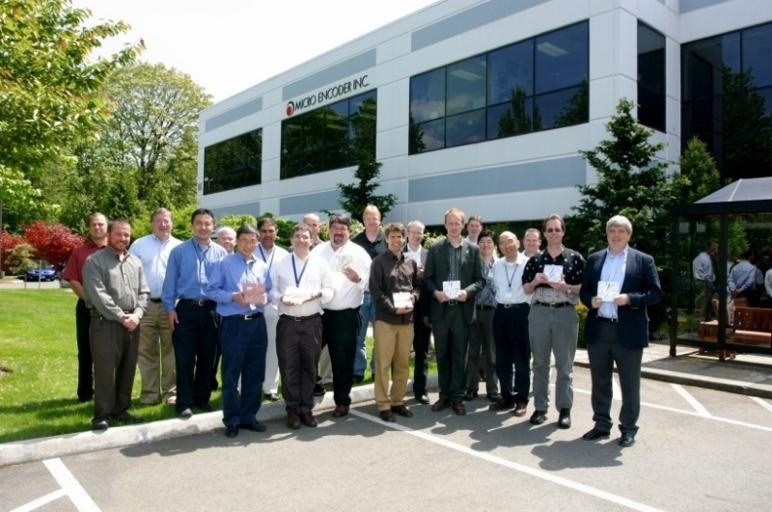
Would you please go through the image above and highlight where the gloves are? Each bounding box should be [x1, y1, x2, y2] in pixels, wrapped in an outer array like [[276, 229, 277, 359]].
[[180, 299, 217, 307]]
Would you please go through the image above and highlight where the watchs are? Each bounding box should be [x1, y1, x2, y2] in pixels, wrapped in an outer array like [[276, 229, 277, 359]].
[[566, 285, 571, 294]]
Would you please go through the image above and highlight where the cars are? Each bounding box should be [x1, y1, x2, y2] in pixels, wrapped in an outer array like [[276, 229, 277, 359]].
[[23, 266, 59, 281]]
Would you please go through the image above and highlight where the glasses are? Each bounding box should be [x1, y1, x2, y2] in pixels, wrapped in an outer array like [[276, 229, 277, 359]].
[[545, 228, 563, 233], [608, 228, 630, 235], [292, 234, 310, 240], [236, 239, 258, 247], [499, 239, 514, 247], [479, 241, 494, 246], [151, 219, 171, 225]]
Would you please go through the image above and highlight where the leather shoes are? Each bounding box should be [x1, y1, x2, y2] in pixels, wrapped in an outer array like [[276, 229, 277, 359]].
[[432, 399, 451, 411], [239, 420, 265, 432], [113, 412, 144, 423], [558, 408, 571, 429], [582, 429, 610, 440], [463, 389, 478, 401], [515, 401, 528, 417], [288, 411, 301, 429], [415, 394, 430, 404], [391, 405, 413, 417], [380, 410, 396, 422], [530, 410, 547, 425], [225, 423, 239, 438], [619, 430, 634, 448], [332, 404, 350, 418], [298, 410, 317, 428], [490, 398, 514, 411], [453, 401, 465, 416], [93, 416, 111, 429], [264, 394, 279, 401]]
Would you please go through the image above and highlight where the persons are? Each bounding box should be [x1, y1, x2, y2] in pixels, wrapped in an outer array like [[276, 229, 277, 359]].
[[309, 213, 372, 418], [425, 205, 543, 416], [269, 224, 336, 429], [238, 217, 289, 401], [579, 214, 662, 448], [205, 222, 266, 438], [160, 208, 228, 417], [521, 212, 587, 428], [212, 227, 238, 390], [81, 219, 150, 430], [127, 208, 185, 405], [62, 212, 109, 402], [352, 203, 390, 384], [691, 242, 772, 357], [370, 219, 433, 418], [288, 212, 334, 396]]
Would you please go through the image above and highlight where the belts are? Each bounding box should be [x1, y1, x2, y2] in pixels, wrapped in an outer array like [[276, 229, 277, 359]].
[[432, 297, 463, 306], [226, 312, 263, 321], [535, 301, 569, 309], [497, 303, 529, 310], [475, 305, 494, 309], [149, 298, 162, 304], [91, 310, 134, 316], [594, 316, 619, 324], [280, 313, 320, 322]]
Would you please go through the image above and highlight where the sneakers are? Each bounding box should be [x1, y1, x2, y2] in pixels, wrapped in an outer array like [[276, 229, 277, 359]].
[[351, 375, 364, 384], [486, 392, 503, 402], [313, 382, 326, 396], [177, 406, 192, 417], [193, 403, 213, 412]]
